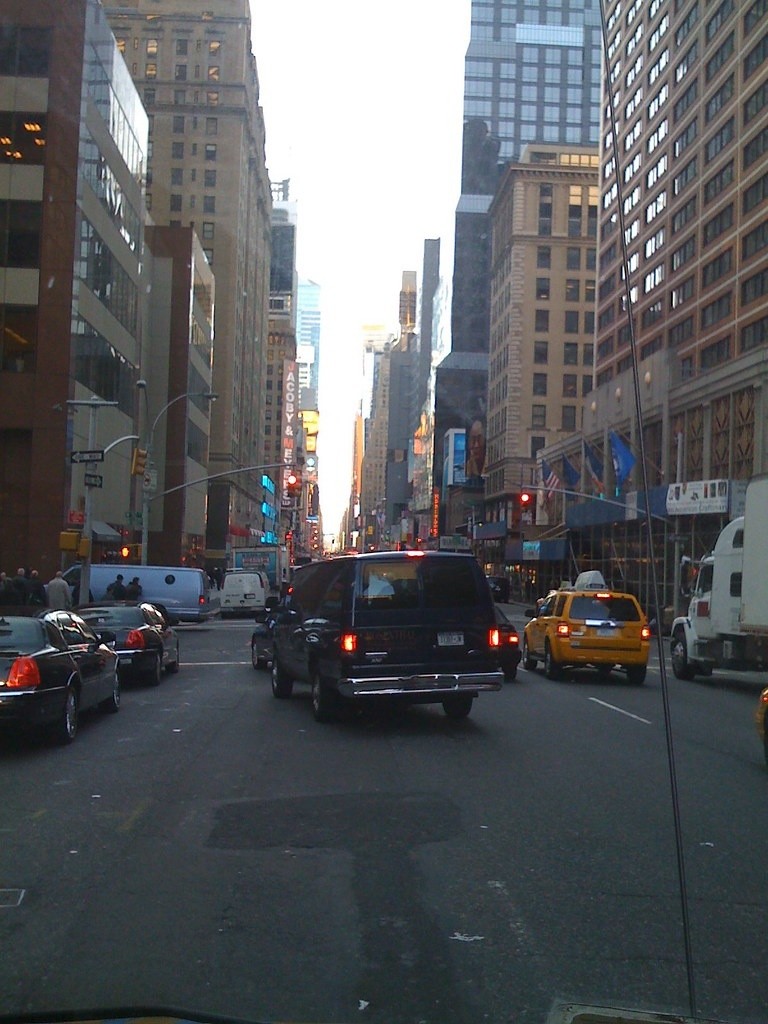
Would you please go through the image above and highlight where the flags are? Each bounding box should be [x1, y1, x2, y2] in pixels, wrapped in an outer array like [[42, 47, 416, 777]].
[[540, 442, 606, 499], [607, 432, 636, 493]]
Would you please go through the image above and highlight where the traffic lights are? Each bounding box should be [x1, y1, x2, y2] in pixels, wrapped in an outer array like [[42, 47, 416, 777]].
[[521, 493, 533, 505], [288, 475, 296, 498], [131, 447, 148, 477], [123, 545, 139, 559]]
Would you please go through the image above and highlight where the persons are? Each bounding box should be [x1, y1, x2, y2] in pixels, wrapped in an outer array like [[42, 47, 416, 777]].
[[537, 598, 547, 609], [467, 420, 487, 477], [0, 567, 47, 609], [47, 572, 72, 609], [71, 579, 94, 606], [207, 565, 226, 591], [101, 575, 142, 602]]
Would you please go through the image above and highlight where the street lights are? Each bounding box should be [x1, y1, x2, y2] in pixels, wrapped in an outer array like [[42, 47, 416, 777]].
[[136, 378, 221, 566]]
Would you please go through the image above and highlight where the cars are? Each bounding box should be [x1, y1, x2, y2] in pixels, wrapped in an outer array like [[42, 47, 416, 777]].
[[494, 605, 523, 682], [523, 570, 651, 686], [265, 551, 504, 724], [0, 605, 122, 742], [70, 600, 180, 687], [486, 573, 510, 605], [251, 609, 275, 668]]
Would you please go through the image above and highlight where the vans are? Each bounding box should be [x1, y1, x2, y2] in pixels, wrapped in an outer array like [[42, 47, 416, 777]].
[[220, 570, 271, 619], [668, 517, 768, 679], [41, 563, 211, 626]]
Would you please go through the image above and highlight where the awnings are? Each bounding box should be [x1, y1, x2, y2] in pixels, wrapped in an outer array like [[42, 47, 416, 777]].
[[89, 520, 124, 544], [229, 526, 268, 538]]
